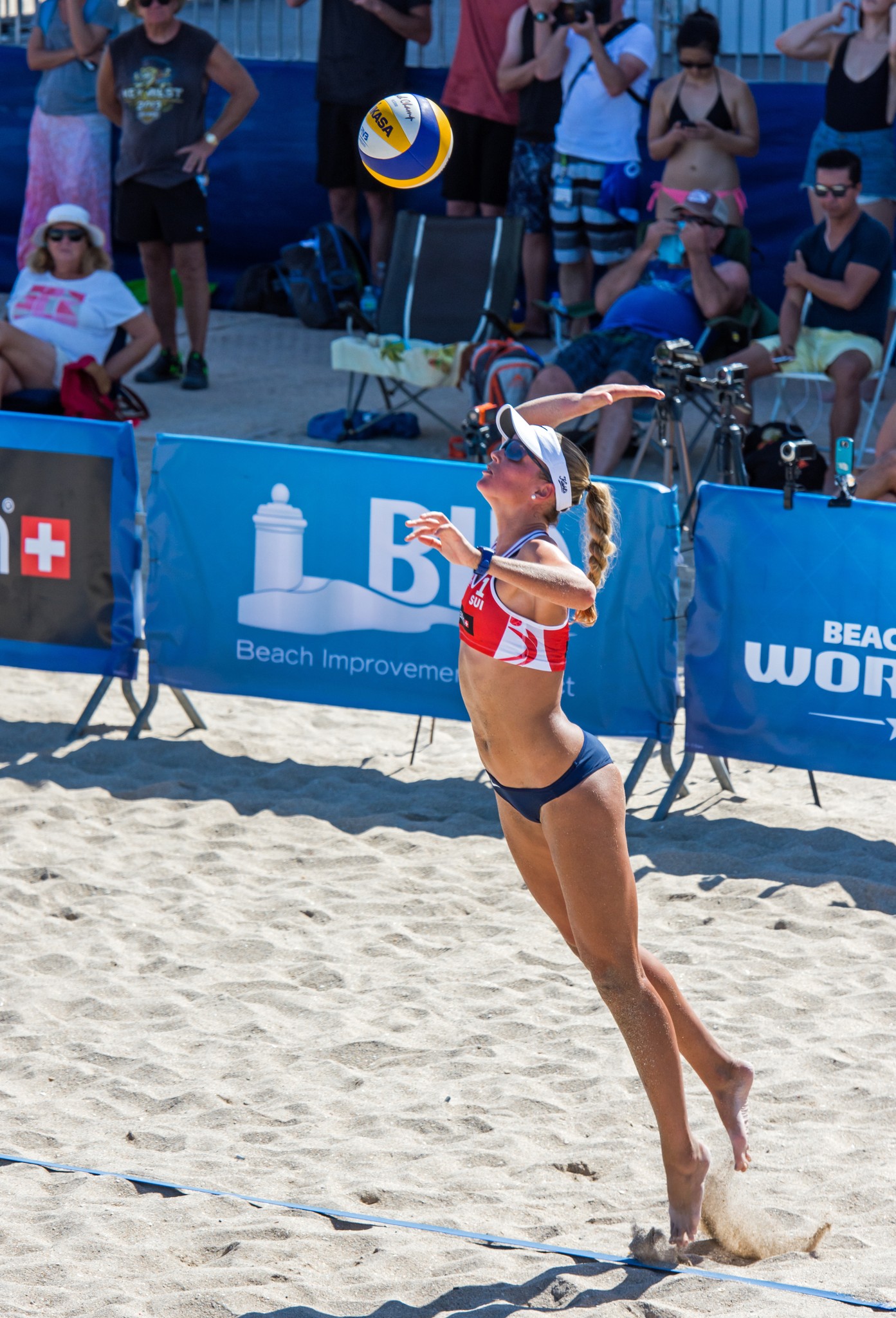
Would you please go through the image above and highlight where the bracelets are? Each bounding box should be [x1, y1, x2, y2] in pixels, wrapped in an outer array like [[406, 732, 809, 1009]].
[[203, 131, 219, 146]]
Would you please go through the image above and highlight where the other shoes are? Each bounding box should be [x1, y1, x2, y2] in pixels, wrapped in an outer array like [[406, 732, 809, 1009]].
[[181, 355, 209, 390], [133, 352, 183, 383]]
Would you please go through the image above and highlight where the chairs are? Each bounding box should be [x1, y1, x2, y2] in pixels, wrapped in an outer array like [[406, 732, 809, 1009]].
[[0, 326, 128, 418], [545, 219, 780, 480], [769, 270, 896, 467], [331, 212, 503, 443]]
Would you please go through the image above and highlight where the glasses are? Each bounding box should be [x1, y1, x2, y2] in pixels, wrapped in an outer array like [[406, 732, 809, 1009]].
[[815, 183, 857, 199], [44, 227, 87, 242], [499, 438, 554, 484], [678, 213, 724, 229], [137, 0, 173, 8], [677, 55, 714, 70]]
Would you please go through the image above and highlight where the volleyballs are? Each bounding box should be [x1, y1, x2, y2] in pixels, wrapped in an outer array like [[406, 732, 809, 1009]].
[[358, 92, 453, 190]]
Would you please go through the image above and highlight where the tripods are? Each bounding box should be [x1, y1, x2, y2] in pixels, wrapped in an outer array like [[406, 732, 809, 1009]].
[[629, 394, 696, 525], [678, 401, 748, 538]]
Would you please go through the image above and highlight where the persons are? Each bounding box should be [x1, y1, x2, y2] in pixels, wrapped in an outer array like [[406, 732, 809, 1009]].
[[405, 385, 753, 1248], [95, 1, 258, 390], [0, 202, 160, 416], [18, 1, 122, 267], [289, 0, 431, 315], [442, 0, 896, 504]]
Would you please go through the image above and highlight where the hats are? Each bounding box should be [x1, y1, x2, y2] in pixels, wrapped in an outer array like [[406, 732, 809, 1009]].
[[670, 188, 729, 226], [33, 202, 105, 247], [495, 404, 572, 513], [126, 0, 186, 18]]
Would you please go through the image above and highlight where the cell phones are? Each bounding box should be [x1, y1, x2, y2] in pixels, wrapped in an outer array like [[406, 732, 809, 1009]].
[[680, 120, 697, 129]]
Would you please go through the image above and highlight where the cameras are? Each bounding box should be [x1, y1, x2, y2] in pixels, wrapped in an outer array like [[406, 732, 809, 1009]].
[[709, 364, 749, 408], [552, 0, 612, 26], [651, 337, 703, 393]]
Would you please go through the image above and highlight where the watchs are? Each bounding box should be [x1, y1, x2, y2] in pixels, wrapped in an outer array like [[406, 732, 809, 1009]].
[[532, 12, 548, 23], [474, 546, 496, 576]]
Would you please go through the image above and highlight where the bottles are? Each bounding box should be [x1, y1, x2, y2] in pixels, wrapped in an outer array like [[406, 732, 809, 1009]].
[[361, 286, 377, 329]]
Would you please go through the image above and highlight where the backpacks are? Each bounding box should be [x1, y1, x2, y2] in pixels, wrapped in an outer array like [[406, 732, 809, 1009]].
[[467, 337, 547, 463], [742, 420, 828, 494], [60, 355, 117, 420]]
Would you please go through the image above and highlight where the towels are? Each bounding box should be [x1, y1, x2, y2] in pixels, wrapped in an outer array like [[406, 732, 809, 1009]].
[[327, 334, 479, 390]]
